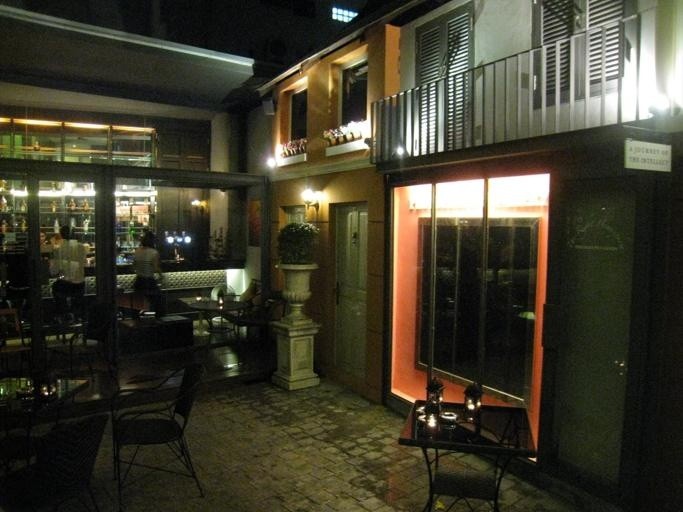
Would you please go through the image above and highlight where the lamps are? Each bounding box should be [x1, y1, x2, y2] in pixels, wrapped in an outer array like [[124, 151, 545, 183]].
[[301, 189, 319, 212], [164, 230, 192, 264]]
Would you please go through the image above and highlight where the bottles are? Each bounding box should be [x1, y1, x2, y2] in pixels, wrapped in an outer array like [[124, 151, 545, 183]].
[[0, 179, 95, 250]]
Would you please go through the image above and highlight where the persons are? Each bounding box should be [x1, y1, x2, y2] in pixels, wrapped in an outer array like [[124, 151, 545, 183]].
[[131, 231, 163, 313], [40, 225, 87, 323]]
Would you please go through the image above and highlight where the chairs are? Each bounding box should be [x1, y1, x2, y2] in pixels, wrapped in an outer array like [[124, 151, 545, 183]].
[[0, 279, 288, 512]]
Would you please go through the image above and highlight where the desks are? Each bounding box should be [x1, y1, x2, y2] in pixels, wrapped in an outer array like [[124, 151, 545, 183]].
[[398, 400, 536, 512]]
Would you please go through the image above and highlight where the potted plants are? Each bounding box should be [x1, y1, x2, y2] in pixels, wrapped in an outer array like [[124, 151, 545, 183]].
[[274, 223, 323, 327], [281, 138, 306, 156], [323, 121, 361, 146]]
